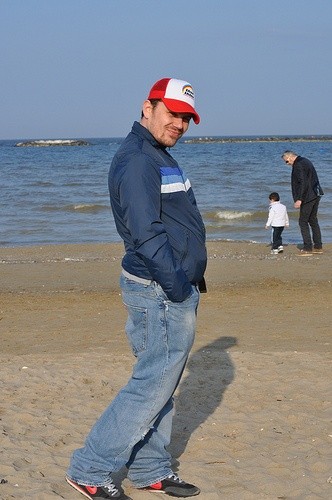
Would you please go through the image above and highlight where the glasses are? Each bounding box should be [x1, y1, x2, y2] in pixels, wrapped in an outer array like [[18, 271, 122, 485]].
[[286, 154, 292, 163]]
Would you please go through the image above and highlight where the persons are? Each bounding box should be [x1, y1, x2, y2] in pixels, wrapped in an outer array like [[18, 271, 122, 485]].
[[264, 192, 290, 255], [282, 150, 325, 256], [65, 78, 209, 500]]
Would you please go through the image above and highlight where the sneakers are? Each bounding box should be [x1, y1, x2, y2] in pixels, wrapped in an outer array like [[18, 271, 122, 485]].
[[65, 476, 133, 500], [296, 249, 313, 256], [312, 249, 323, 253], [270, 249, 278, 254], [278, 245, 283, 253], [136, 473, 200, 498]]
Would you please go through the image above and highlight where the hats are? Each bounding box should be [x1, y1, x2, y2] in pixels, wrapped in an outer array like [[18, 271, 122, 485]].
[[148, 77, 200, 124]]
